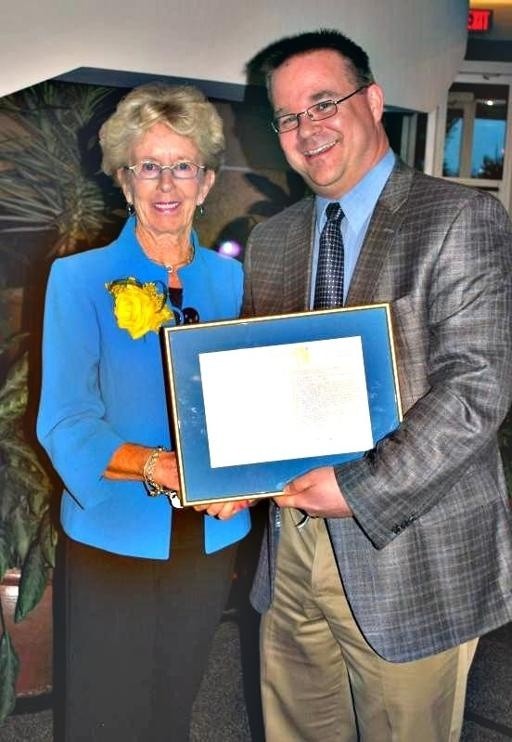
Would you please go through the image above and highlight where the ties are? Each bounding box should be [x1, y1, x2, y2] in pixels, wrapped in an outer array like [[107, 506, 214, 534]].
[[313, 202, 346, 311]]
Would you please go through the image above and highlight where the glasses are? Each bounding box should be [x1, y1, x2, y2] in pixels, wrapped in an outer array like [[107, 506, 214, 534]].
[[270, 87, 366, 133], [125, 163, 207, 178]]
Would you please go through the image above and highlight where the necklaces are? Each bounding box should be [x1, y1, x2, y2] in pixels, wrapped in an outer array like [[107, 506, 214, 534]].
[[148, 256, 192, 277]]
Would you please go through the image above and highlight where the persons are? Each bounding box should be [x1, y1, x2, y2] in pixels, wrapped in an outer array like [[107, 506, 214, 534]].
[[190, 26, 512, 742], [34, 79, 254, 742]]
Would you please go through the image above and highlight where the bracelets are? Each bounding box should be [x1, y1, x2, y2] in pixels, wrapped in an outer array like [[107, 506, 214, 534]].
[[147, 446, 170, 496], [143, 448, 155, 496]]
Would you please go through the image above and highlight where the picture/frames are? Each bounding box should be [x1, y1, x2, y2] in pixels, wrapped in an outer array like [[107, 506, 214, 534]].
[[164, 301, 403, 510]]
